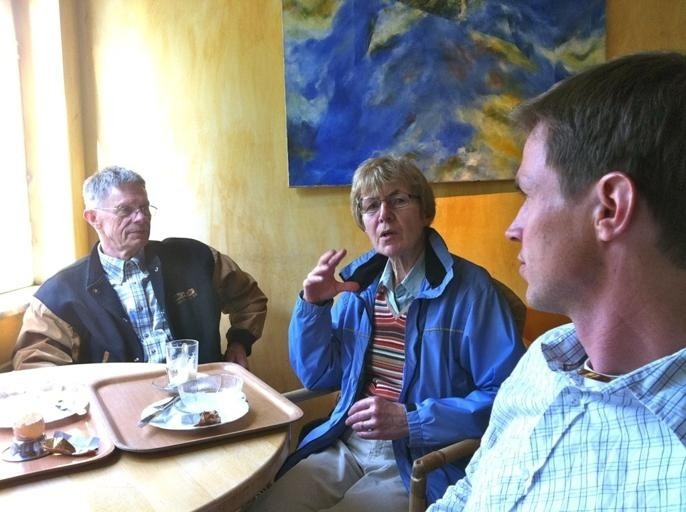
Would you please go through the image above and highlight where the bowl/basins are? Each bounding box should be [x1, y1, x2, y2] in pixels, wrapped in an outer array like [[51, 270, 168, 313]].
[[178, 374, 243, 410]]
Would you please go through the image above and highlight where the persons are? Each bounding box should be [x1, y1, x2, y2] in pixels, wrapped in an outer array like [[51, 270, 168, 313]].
[[426, 48, 686, 512], [241, 146, 526, 510], [8, 165, 269, 370]]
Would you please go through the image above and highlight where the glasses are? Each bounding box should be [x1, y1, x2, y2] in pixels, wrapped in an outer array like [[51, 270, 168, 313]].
[[94, 202, 159, 218], [358, 191, 421, 215]]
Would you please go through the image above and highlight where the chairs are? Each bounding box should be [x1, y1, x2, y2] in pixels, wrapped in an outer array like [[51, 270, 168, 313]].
[[282, 276, 527, 511]]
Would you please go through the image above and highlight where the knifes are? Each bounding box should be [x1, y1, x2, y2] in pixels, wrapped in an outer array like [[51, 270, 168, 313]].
[[138, 399, 180, 430]]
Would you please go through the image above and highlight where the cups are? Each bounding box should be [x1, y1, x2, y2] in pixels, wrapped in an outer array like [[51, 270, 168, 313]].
[[166, 339, 198, 387]]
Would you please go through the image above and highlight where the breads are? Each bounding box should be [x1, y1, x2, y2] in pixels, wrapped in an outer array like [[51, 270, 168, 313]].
[[13, 413, 44, 440], [193, 410, 222, 427]]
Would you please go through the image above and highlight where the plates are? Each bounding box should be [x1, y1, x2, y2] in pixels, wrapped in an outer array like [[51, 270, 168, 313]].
[[0, 390, 91, 429], [152, 372, 210, 396], [141, 394, 249, 432]]
